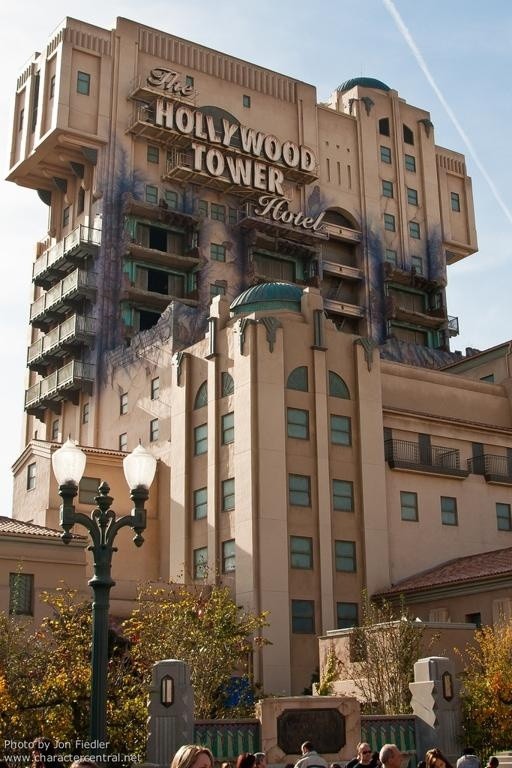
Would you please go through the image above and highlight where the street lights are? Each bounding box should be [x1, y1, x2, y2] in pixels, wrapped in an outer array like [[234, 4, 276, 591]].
[[52, 434, 158, 764]]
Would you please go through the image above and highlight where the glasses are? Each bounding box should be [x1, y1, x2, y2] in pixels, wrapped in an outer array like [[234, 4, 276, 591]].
[[362, 750, 372, 755]]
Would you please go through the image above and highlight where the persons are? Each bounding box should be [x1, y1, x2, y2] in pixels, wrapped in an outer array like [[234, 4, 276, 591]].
[[27, 736, 500, 768]]
